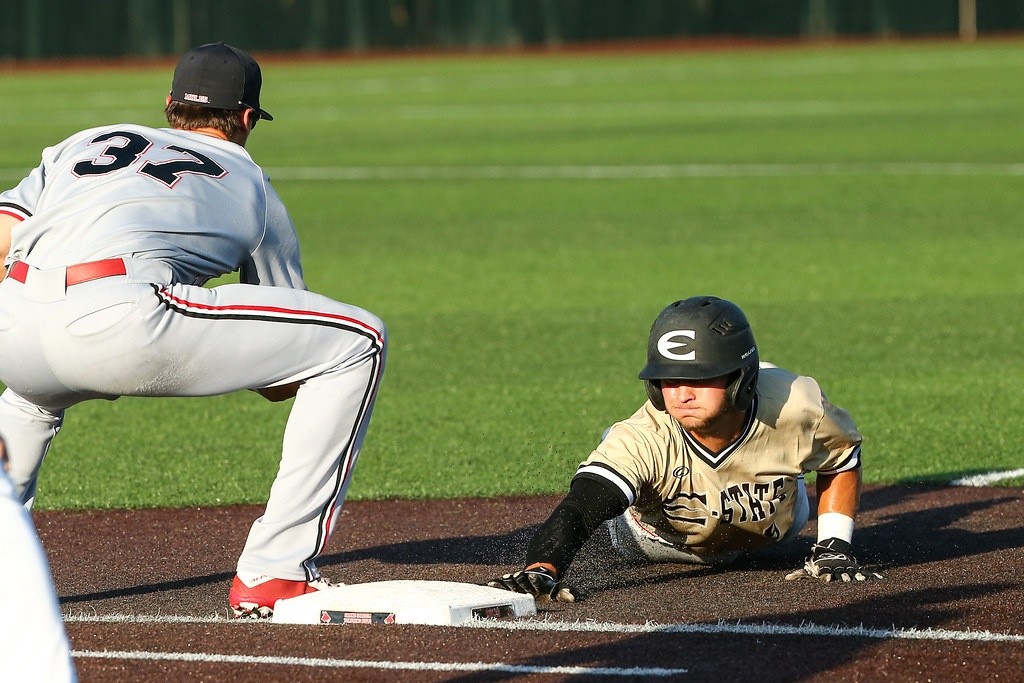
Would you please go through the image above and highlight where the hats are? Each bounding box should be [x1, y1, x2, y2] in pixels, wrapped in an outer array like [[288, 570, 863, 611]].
[[169, 40, 274, 121]]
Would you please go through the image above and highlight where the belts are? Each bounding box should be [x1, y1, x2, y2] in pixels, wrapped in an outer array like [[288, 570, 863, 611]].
[[8, 257, 126, 284]]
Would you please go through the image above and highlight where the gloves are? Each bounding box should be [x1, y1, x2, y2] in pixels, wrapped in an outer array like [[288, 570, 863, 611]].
[[786, 537, 883, 582], [488, 566, 577, 602]]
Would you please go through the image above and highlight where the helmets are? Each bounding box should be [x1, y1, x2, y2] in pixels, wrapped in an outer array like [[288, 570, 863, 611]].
[[638, 296, 758, 413]]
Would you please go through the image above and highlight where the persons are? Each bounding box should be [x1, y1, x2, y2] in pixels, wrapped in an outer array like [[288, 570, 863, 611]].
[[0, 40, 389, 618], [487, 295, 886, 605]]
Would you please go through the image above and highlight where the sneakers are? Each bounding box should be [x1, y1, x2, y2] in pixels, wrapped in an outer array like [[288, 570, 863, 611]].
[[230, 574, 348, 618]]
